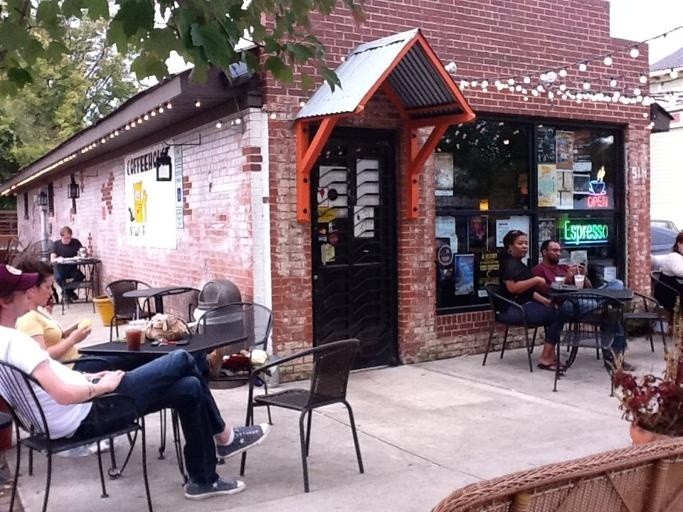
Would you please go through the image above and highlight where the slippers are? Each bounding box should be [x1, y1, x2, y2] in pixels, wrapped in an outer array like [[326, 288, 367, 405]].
[[538, 359, 568, 371]]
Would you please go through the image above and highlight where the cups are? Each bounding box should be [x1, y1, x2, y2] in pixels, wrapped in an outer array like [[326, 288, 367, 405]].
[[122, 328, 143, 352], [591, 180, 604, 194], [129, 320, 147, 344], [573, 274, 585, 289], [554, 276, 565, 285], [79, 246, 86, 257]]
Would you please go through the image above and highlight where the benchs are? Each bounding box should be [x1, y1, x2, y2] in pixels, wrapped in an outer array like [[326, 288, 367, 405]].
[[431, 437, 682, 511]]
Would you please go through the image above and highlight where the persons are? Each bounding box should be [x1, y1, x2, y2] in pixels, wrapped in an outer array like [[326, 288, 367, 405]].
[[494, 230, 566, 372], [653, 228, 682, 315], [50, 226, 88, 307], [0, 264, 271, 501], [14, 257, 142, 372], [533, 240, 635, 371]]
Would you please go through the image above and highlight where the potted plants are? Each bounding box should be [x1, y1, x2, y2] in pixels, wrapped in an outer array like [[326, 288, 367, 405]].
[[93, 296, 127, 327], [604, 319, 683, 448]]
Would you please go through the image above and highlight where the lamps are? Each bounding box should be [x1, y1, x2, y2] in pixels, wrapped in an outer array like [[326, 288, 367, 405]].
[[155, 134, 202, 181], [66, 170, 98, 199], [36, 184, 62, 205]]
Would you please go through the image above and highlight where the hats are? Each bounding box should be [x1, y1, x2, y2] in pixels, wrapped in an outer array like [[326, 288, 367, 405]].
[[0, 264, 39, 291]]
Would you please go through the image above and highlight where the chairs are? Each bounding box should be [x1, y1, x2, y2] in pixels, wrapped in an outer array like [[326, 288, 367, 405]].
[[613, 288, 668, 356], [105, 280, 157, 343], [482, 283, 540, 373], [0, 359, 153, 511], [552, 290, 625, 397], [188, 300, 275, 426], [651, 270, 683, 335], [187, 277, 244, 340], [29, 239, 101, 316], [239, 337, 365, 493]]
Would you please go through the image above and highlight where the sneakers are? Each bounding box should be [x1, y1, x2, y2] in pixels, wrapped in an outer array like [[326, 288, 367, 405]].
[[184, 478, 246, 501], [216, 422, 270, 458]]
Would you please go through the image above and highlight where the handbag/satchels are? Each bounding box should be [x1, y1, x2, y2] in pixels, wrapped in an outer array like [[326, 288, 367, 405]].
[[145, 313, 189, 342], [588, 265, 605, 288]]
